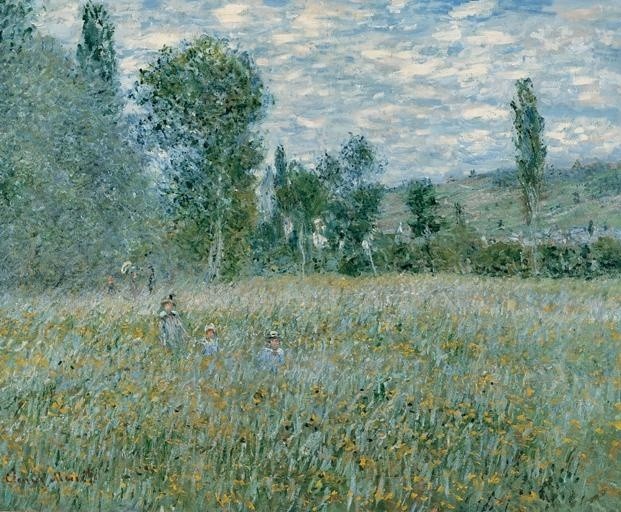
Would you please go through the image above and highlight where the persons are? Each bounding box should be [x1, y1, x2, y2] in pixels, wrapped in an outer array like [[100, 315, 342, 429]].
[[158, 297, 189, 345], [257, 328, 288, 366], [105, 271, 140, 293], [195, 323, 220, 356]]
